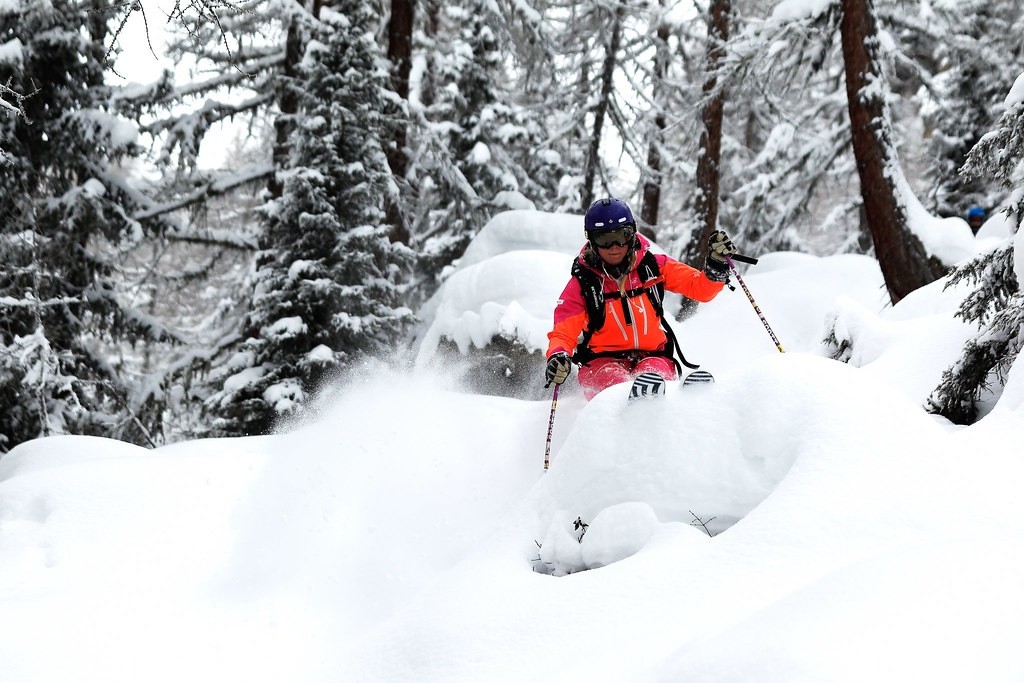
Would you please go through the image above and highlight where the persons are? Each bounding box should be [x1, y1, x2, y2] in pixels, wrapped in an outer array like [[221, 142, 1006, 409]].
[[545, 197, 737, 405], [967, 207, 985, 236]]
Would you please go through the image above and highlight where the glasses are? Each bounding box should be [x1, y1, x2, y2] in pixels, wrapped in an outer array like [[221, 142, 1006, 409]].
[[588, 228, 633, 248]]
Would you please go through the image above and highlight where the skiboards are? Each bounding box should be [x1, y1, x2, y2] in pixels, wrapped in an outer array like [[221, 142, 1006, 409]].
[[626, 372, 667, 403], [681, 370, 715, 386]]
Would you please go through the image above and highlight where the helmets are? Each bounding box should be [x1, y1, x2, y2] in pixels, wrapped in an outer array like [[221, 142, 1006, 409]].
[[584, 198, 636, 233]]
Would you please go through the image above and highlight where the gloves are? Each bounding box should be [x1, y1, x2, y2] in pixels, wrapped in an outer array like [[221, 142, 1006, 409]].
[[704, 231, 737, 282], [545, 351, 571, 383]]
